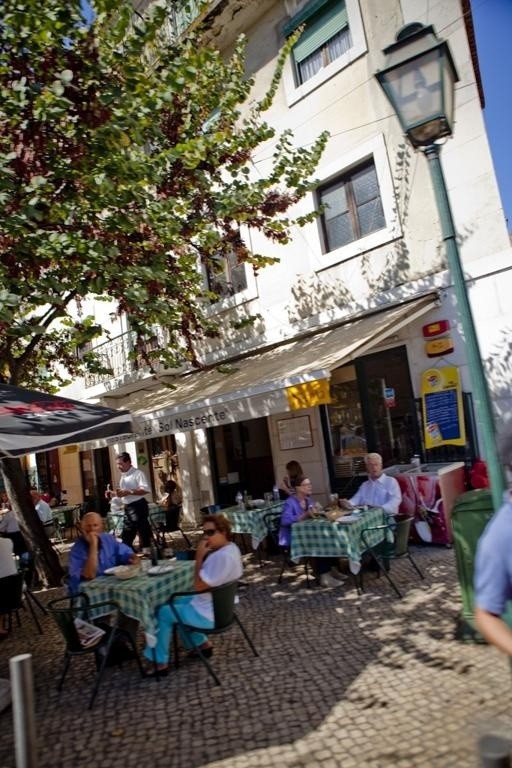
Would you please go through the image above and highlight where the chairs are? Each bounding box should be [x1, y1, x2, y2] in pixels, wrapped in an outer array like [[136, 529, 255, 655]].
[[170, 576, 259, 684], [0, 571, 47, 635], [150, 505, 193, 549], [62, 571, 82, 592], [201, 503, 248, 553], [358, 512, 425, 598], [264, 512, 332, 588], [48, 593, 146, 710], [42, 517, 68, 556]]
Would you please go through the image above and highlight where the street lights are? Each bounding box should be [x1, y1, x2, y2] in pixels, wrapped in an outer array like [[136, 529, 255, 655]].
[[373, 21, 505, 510]]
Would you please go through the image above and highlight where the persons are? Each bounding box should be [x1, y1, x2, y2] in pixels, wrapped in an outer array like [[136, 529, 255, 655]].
[[283, 458, 308, 503], [0, 536, 18, 641], [66, 512, 139, 671], [338, 452, 405, 574], [278, 475, 350, 588], [157, 469, 183, 531], [28, 490, 54, 528], [475, 415, 512, 655], [111, 452, 152, 554], [138, 515, 243, 680], [0, 499, 22, 534]]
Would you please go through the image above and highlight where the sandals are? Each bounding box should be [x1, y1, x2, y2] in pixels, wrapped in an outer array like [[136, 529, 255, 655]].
[[144, 663, 169, 678], [183, 640, 214, 660]]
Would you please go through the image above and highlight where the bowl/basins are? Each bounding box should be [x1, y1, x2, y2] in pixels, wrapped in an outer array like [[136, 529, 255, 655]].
[[114, 565, 142, 579]]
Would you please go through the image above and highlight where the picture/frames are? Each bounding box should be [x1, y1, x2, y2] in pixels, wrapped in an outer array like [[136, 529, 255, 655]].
[[275, 413, 315, 450]]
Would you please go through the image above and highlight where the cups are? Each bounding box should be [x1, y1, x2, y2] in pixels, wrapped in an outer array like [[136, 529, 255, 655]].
[[327, 494, 337, 509], [244, 495, 253, 507], [116, 488, 122, 497], [309, 504, 320, 519], [140, 557, 149, 572], [327, 511, 337, 522], [265, 492, 274, 507]]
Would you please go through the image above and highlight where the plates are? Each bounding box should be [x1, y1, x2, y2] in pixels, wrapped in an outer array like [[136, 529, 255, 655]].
[[337, 516, 359, 524], [347, 504, 371, 509], [148, 565, 175, 575], [104, 565, 125, 575]]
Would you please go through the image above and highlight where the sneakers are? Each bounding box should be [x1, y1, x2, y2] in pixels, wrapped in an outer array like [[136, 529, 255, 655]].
[[333, 571, 348, 580], [320, 576, 344, 587]]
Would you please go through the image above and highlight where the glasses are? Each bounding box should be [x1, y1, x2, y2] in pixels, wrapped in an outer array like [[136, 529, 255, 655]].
[[202, 528, 217, 536], [301, 482, 312, 486]]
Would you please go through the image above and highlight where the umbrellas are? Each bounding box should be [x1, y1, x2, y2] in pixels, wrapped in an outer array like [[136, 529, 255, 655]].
[[0, 383, 136, 459]]
